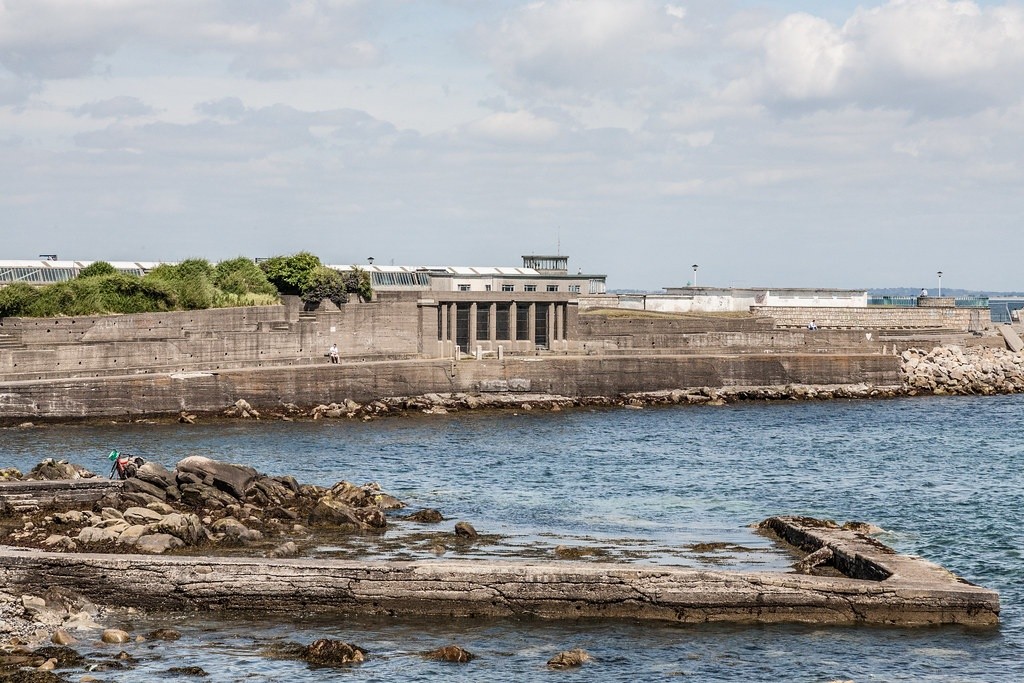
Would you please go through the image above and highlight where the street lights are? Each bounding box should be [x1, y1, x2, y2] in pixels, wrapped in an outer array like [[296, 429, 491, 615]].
[[937, 270, 943, 297], [692, 263, 699, 287]]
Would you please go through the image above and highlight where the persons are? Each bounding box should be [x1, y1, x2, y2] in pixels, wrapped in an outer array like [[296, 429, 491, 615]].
[[330, 344, 338, 363], [809, 320, 818, 330], [920, 288, 928, 297]]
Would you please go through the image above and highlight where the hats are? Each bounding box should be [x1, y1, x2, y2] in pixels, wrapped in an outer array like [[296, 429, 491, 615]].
[[108, 451, 119, 462]]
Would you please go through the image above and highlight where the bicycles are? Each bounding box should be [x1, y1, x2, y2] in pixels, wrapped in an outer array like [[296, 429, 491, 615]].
[[108, 448, 133, 480]]
